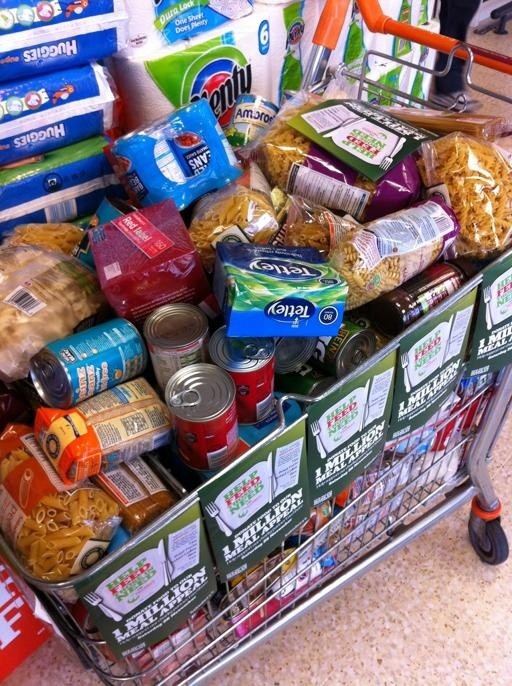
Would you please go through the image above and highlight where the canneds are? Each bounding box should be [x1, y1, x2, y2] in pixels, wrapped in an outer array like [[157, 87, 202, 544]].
[[28, 259, 466, 473], [226, 93, 279, 151]]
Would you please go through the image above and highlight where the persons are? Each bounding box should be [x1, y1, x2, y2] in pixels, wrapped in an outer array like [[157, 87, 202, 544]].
[[430, 0, 485, 110]]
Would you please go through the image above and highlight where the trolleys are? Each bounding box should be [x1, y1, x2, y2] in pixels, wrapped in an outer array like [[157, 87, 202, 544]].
[[0, 0, 512, 685]]
